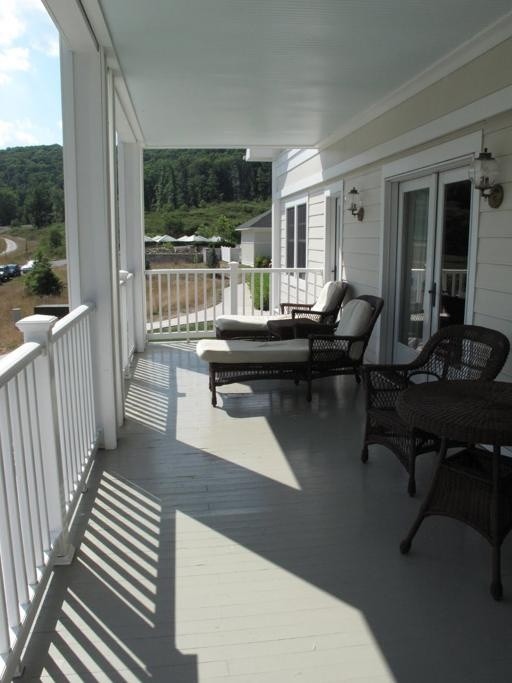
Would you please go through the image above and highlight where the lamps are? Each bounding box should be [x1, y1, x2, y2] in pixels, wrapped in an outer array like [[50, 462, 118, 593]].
[[473, 147, 504, 209], [346, 187, 364, 221]]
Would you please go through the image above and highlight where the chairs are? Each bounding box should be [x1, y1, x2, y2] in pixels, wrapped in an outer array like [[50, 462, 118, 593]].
[[215, 281, 350, 372], [359, 325, 512, 497], [440, 294, 465, 346], [196, 294, 384, 407]]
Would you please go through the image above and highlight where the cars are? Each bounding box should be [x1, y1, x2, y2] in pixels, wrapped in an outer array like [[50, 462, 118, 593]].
[[0, 260, 38, 282]]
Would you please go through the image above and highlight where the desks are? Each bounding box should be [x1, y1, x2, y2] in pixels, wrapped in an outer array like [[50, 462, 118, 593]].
[[395, 379, 512, 602]]
